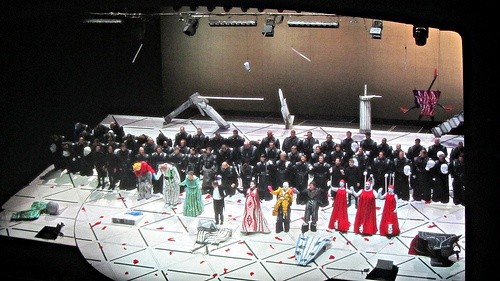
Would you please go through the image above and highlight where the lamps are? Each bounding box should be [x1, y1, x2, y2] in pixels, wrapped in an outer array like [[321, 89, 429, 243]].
[[413, 26, 429, 45], [179, 12, 383, 41]]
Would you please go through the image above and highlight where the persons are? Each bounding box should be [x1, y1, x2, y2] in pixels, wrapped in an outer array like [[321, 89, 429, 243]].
[[50, 122, 465, 237], [199, 180, 235, 224]]
